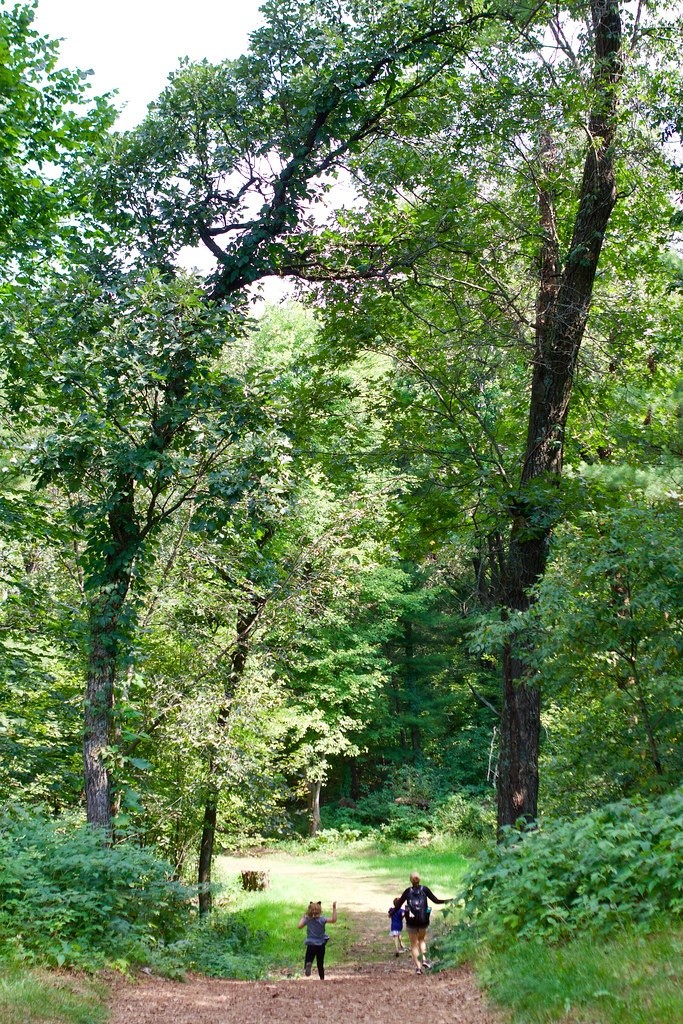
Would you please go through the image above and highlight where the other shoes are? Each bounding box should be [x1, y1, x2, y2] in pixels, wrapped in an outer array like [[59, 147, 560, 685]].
[[305, 962, 312, 977], [416, 968, 423, 975], [396, 952, 399, 958], [422, 960, 430, 969]]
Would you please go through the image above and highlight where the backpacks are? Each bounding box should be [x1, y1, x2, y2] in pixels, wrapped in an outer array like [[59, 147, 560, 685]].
[[405, 886, 428, 926]]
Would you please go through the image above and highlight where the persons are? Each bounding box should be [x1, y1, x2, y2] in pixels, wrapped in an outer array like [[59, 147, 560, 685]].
[[388, 899, 405, 957], [299, 901, 336, 980], [389, 873, 453, 975]]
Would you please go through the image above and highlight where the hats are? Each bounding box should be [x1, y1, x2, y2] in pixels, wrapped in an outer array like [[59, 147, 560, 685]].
[[410, 872, 420, 883]]
